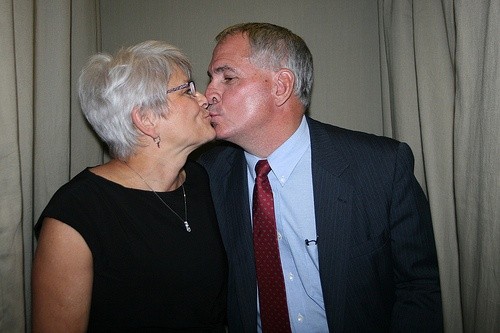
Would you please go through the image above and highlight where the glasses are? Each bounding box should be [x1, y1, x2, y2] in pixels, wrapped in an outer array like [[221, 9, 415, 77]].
[[135, 80, 196, 106]]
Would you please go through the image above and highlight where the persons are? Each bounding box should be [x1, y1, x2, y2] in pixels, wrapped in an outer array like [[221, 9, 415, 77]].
[[206, 22, 445, 333], [31, 39, 229, 333]]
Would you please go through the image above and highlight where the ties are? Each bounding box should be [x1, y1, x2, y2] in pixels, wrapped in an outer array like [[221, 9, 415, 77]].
[[253, 159, 292, 333]]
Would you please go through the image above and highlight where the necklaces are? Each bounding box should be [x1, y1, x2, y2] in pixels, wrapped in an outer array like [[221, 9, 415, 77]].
[[126, 161, 191, 232]]
